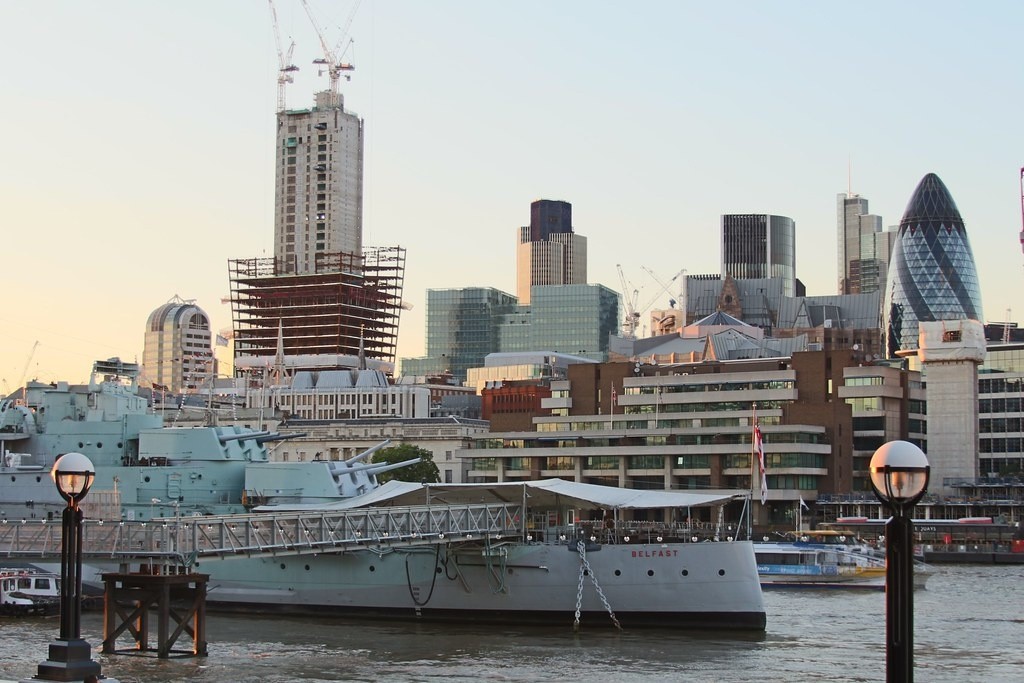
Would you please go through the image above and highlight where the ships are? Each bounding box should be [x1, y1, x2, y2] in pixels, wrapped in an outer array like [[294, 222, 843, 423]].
[[0, 357, 769, 630]]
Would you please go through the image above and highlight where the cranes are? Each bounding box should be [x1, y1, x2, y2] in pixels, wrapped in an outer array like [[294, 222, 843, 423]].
[[267, 0, 361, 110], [618, 263, 688, 340]]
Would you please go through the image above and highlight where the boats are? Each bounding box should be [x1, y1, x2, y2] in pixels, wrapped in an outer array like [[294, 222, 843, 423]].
[[0, 567, 62, 618], [753, 528, 939, 589]]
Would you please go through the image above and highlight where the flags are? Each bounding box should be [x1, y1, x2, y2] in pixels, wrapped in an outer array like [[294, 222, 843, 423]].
[[753, 407, 768, 505], [265, 360, 272, 372], [215, 334, 228, 347], [800, 497, 809, 509]]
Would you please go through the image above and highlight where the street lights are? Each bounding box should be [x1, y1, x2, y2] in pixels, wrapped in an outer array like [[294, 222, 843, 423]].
[[32, 451, 109, 683], [867, 439, 930, 683]]
